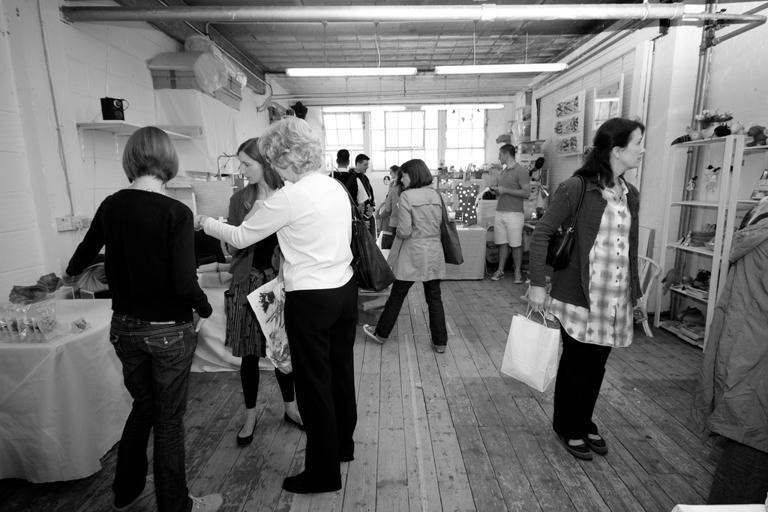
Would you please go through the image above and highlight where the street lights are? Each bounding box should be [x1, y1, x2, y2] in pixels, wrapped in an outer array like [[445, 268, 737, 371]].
[[440, 221, 463, 265], [501, 314, 563, 393], [350, 221, 395, 291], [246, 276, 293, 374], [546, 227, 575, 270]]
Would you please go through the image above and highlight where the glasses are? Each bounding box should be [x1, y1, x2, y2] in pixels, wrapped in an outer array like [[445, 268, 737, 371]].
[[285, 20, 418, 77], [322, 76, 407, 113], [420, 74, 504, 111], [434, 19, 569, 74]]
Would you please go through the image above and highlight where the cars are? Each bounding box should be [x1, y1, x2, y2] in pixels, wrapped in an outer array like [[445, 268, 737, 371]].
[[0, 304, 64, 343]]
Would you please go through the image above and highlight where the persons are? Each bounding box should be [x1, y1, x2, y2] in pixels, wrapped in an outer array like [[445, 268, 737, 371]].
[[490, 143, 531, 284], [363, 160, 447, 353], [65, 126, 224, 512], [191, 116, 360, 494], [528, 117, 647, 462], [687, 196, 767, 506], [225, 136, 307, 448], [328, 149, 402, 245]]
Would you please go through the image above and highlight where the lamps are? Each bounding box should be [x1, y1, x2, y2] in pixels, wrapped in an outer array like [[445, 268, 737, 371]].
[[633, 255, 662, 338]]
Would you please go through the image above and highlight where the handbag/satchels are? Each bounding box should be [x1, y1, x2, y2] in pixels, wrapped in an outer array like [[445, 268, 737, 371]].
[[198, 214, 204, 228]]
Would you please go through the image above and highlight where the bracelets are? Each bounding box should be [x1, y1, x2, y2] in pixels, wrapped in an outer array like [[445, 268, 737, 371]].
[[703, 241, 715, 251]]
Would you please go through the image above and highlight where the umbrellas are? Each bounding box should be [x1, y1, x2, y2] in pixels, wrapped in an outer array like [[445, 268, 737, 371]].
[[237, 418, 257, 447], [284, 412, 305, 431], [283, 469, 341, 494]]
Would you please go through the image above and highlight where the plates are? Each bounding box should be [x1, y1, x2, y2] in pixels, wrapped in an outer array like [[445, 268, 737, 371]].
[[653, 134, 768, 355]]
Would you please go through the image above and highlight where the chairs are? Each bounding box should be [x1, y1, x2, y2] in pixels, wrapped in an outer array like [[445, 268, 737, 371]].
[[564, 439, 593, 459], [513, 271, 524, 284], [188, 493, 223, 512], [363, 324, 387, 344], [430, 338, 445, 353], [112, 473, 155, 511], [586, 433, 608, 454], [491, 270, 504, 281]]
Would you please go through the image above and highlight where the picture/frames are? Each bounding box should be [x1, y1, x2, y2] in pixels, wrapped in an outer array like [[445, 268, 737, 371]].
[[524, 221, 562, 235], [376, 224, 486, 281]]
